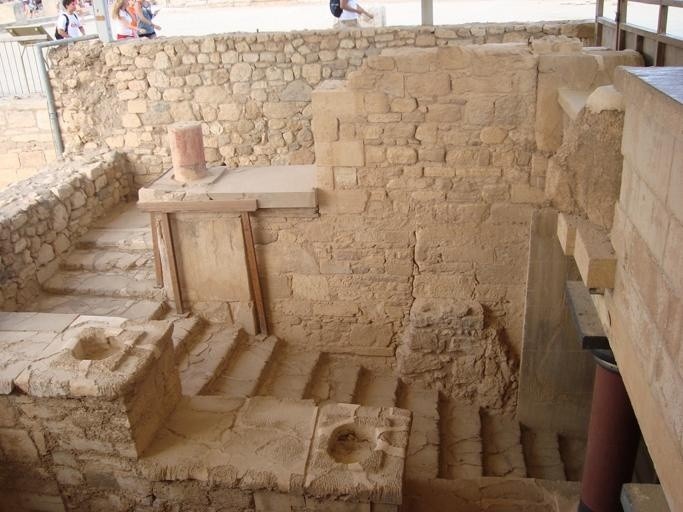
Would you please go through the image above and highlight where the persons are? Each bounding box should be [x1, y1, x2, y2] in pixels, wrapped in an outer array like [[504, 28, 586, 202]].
[[338, 0, 374, 25], [12, 0, 42, 20], [111, 0, 161, 40], [57, 0, 92, 39]]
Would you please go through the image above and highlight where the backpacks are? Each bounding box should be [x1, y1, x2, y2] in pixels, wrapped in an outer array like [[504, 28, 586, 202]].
[[55, 14, 77, 39], [330, 0, 342, 16]]
